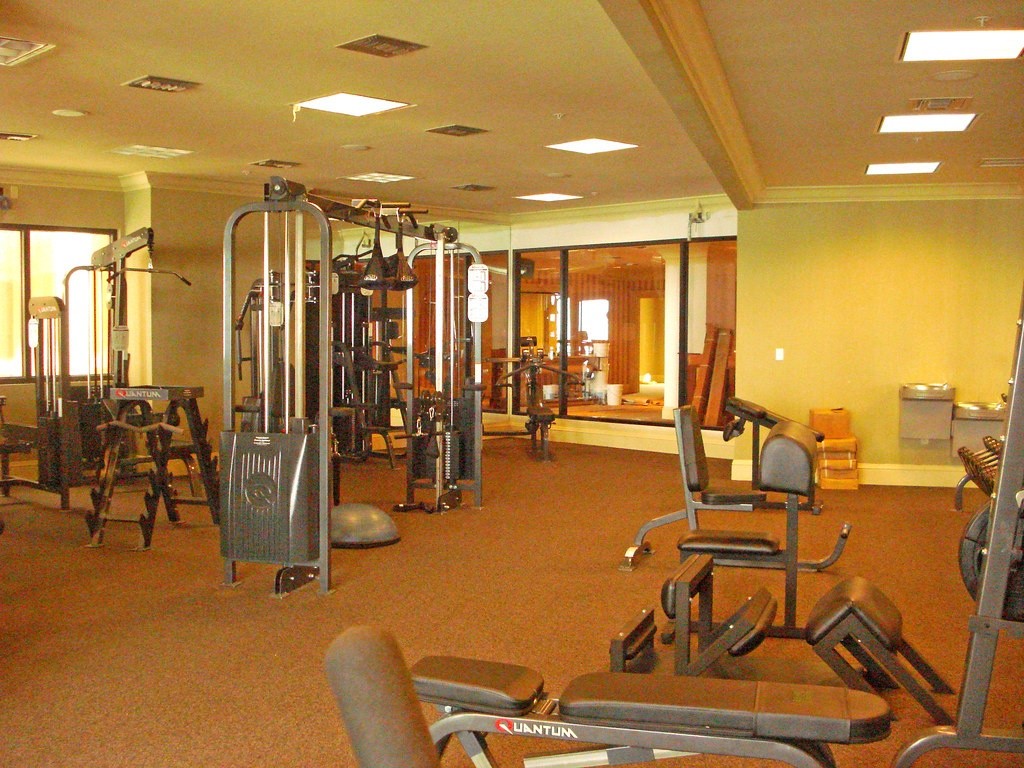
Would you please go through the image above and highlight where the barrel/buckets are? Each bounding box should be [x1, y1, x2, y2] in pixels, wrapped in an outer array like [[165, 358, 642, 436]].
[[618, 394, 619, 395], [594, 343, 610, 358], [542, 384, 559, 401]]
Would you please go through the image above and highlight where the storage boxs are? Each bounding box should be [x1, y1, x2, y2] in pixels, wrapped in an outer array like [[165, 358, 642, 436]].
[[705, 329, 734, 426], [820, 469, 858, 479], [819, 459, 857, 470], [822, 479, 858, 490], [817, 452, 855, 460], [810, 407, 852, 439], [816, 434, 858, 452], [691, 321, 719, 424]]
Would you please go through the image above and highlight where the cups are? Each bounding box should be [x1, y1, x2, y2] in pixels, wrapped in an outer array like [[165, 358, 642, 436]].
[[607, 384, 623, 406]]
